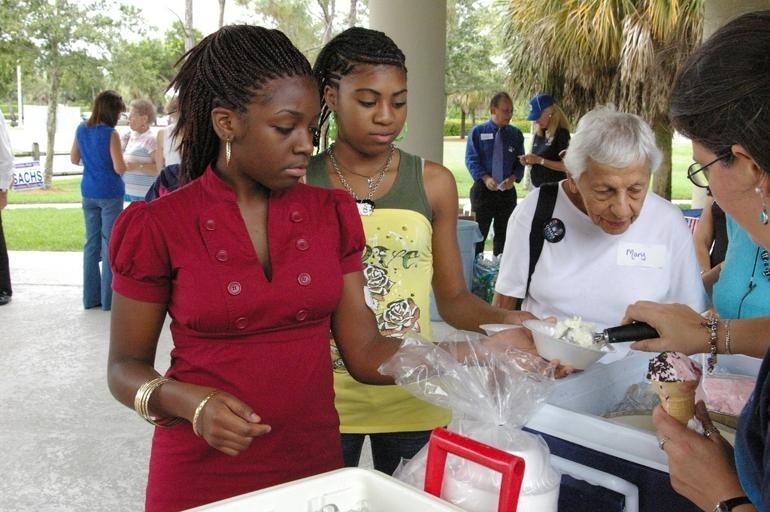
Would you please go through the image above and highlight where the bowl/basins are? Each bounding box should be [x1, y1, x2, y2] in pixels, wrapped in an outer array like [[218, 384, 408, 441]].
[[457, 429, 561, 509], [521, 319, 613, 372]]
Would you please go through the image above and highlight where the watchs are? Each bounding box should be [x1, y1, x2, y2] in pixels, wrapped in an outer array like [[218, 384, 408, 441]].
[[139, 163, 144, 171], [540, 158, 545, 165]]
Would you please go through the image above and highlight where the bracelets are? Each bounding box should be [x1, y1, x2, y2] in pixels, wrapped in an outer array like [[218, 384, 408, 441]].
[[699, 269, 707, 276], [191, 389, 218, 438], [724, 320, 732, 355], [701, 312, 718, 373], [133, 378, 184, 429], [712, 496, 752, 512]]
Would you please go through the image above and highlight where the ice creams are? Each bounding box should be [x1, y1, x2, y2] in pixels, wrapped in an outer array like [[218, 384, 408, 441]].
[[644, 350, 704, 430]]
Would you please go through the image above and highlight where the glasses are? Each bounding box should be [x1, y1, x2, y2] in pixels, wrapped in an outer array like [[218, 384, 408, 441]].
[[687, 151, 733, 188]]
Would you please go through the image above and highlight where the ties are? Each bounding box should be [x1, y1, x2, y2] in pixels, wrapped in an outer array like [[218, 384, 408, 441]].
[[492, 128, 504, 184]]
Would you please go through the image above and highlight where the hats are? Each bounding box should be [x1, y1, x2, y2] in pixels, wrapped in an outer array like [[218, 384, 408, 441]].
[[527, 94, 556, 121]]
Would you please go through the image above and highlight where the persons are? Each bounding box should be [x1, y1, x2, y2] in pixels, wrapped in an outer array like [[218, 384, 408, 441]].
[[107, 24, 573, 512], [465, 93, 525, 258], [490, 102, 709, 364], [120, 100, 157, 211], [70, 90, 127, 310], [517, 93, 570, 187], [297, 27, 557, 477], [145, 164, 182, 203], [0, 111, 15, 304], [156, 95, 184, 176], [621, 8, 769, 512], [692, 194, 728, 294]]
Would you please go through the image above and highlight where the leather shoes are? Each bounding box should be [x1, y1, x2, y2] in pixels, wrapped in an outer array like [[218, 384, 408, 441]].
[[0, 289, 12, 305]]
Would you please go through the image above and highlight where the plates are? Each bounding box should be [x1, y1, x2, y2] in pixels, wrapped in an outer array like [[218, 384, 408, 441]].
[[479, 323, 523, 335]]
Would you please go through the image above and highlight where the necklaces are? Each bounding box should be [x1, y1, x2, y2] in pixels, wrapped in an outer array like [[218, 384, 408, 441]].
[[327, 142, 395, 217], [337, 160, 378, 189]]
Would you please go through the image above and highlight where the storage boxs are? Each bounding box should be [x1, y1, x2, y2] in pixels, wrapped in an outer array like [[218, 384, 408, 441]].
[[516, 356, 748, 511], [179, 466, 468, 512]]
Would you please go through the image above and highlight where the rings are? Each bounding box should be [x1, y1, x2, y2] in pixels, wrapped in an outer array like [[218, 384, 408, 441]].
[[705, 426, 718, 437], [526, 160, 528, 163], [658, 438, 668, 449]]
